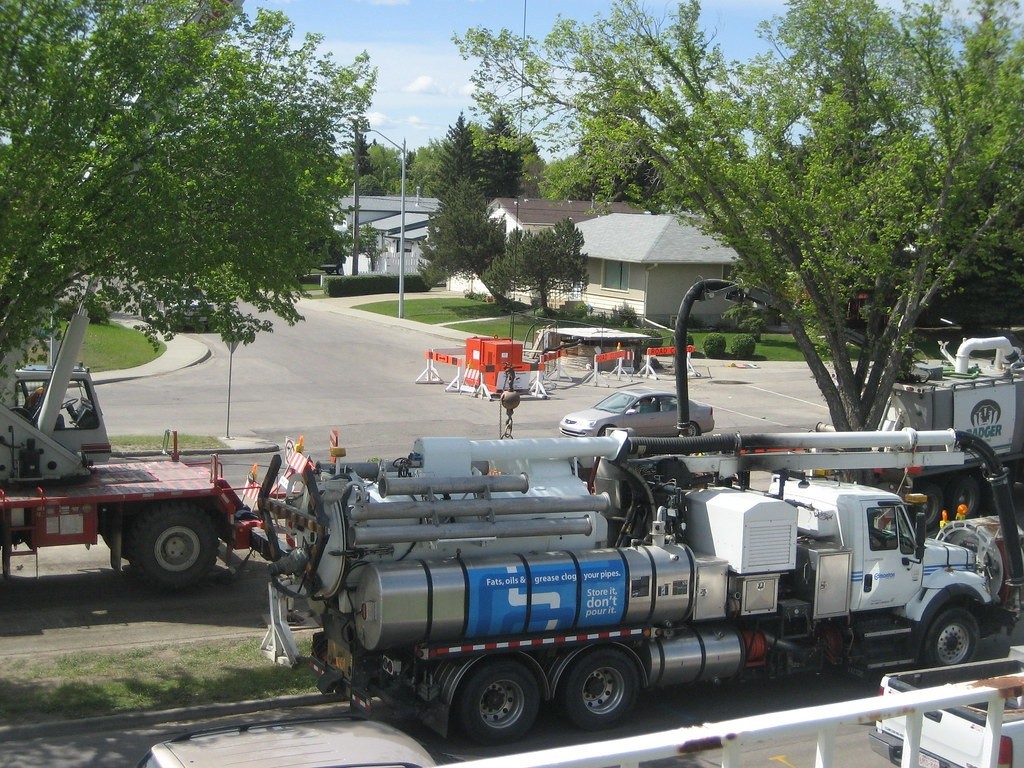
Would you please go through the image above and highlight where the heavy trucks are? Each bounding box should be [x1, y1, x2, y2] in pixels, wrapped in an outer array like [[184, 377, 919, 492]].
[[255, 277, 1023, 748]]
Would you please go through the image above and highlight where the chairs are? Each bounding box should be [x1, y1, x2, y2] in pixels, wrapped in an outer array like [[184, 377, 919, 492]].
[[633, 398, 677, 414]]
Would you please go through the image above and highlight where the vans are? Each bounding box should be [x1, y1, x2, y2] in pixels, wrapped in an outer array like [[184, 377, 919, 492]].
[[133, 708, 437, 768]]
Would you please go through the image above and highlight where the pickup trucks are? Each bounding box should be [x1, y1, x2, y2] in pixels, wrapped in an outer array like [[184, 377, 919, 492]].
[[864, 645, 1023, 767]]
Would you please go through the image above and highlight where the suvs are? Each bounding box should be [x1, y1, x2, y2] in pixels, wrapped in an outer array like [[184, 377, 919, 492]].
[[140, 282, 217, 333]]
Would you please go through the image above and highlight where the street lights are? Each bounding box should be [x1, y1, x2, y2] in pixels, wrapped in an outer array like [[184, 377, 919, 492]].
[[359, 128, 406, 319]]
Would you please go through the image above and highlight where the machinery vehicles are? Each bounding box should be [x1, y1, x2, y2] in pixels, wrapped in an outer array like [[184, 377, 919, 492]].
[[0, 273, 316, 597]]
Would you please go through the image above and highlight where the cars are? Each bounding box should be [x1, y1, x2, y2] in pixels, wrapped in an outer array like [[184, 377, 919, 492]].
[[558, 387, 715, 439]]
